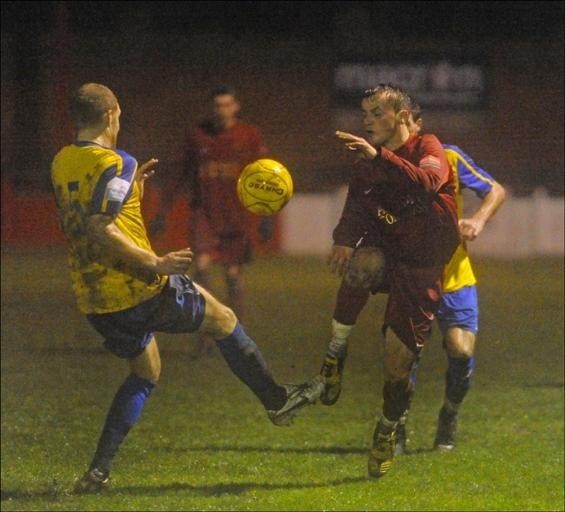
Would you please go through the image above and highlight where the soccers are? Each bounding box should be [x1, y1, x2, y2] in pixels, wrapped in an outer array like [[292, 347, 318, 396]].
[[237, 160, 293, 218]]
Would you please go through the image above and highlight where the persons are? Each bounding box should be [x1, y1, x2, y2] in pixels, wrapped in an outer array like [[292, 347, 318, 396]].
[[44, 82, 324, 495], [316, 80, 462, 479], [147, 86, 265, 358], [389, 93, 507, 458]]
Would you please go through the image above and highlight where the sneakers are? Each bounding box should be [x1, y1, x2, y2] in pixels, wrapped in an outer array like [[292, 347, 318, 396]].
[[433, 406, 458, 453], [393, 423, 407, 458], [319, 340, 349, 405], [266, 373, 327, 427], [74, 468, 111, 495], [367, 428, 398, 479]]
